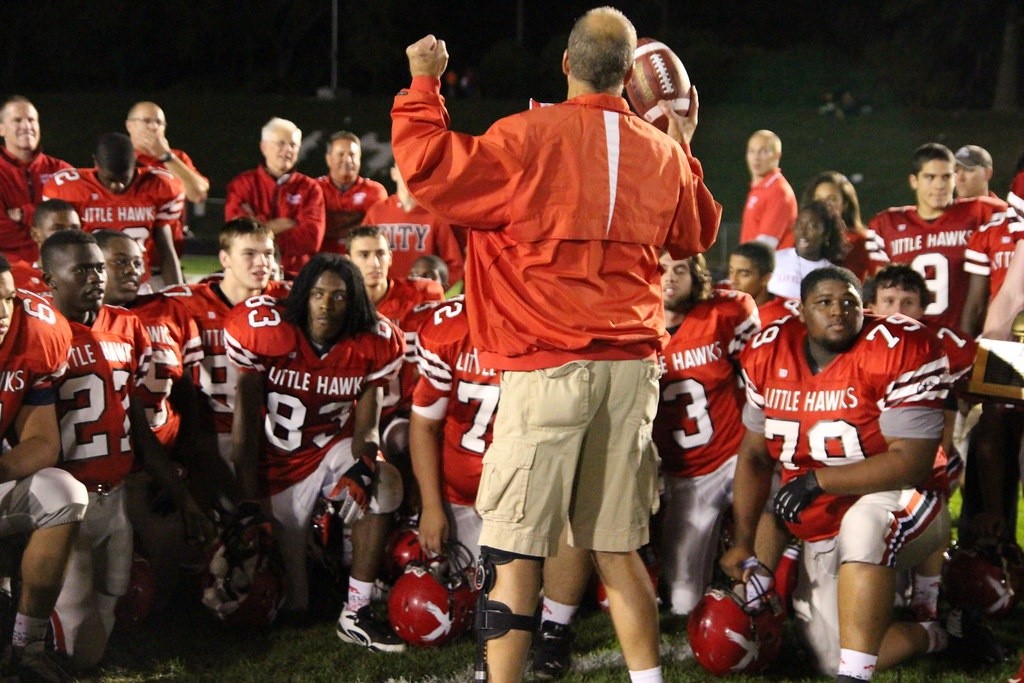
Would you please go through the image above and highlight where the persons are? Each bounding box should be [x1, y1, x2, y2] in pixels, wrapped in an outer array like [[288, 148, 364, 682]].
[[0, 98, 1024, 683], [389, 5, 723, 683]]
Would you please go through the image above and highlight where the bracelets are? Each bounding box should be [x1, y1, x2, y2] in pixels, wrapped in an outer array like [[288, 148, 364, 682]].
[[158, 152, 172, 162]]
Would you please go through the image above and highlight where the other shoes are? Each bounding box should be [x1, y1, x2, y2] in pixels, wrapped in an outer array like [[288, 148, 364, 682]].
[[902, 602, 939, 624], [945, 603, 1000, 676]]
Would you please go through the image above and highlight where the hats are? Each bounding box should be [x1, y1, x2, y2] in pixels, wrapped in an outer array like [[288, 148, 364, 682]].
[[953, 145, 993, 169]]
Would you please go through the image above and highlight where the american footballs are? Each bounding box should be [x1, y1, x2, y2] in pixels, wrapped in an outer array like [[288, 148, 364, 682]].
[[626, 36, 691, 136]]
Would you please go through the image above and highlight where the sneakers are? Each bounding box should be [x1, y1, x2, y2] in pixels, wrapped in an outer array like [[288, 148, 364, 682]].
[[533, 620, 577, 682], [335, 601, 408, 654], [7, 638, 75, 683]]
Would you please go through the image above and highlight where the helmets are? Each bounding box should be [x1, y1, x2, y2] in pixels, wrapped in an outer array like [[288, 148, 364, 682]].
[[686, 582, 784, 675], [384, 524, 450, 576], [114, 554, 154, 627], [596, 559, 662, 616], [389, 563, 477, 648], [943, 535, 1024, 618], [200, 540, 287, 631]]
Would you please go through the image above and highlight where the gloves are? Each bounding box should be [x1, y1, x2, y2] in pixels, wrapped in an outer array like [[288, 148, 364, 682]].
[[777, 472, 824, 524], [330, 455, 379, 524]]
